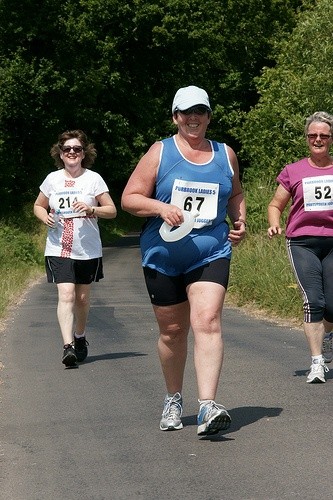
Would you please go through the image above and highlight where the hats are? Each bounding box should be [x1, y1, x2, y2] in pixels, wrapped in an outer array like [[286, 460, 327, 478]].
[[172, 85, 212, 114]]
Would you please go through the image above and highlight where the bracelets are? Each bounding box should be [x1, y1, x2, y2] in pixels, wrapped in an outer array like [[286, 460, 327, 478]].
[[233, 219, 247, 228], [85, 206, 95, 215]]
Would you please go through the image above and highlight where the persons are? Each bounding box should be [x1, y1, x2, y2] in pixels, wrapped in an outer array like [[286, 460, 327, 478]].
[[266, 109, 333, 387], [32, 130, 117, 368], [120, 83, 247, 436]]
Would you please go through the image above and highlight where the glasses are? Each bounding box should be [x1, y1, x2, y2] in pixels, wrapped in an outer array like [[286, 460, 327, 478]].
[[306, 134, 331, 139], [181, 106, 208, 115], [61, 147, 84, 152]]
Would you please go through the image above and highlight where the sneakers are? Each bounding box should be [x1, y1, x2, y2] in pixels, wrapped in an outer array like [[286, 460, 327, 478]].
[[197, 399, 231, 436], [321, 332, 333, 362], [73, 331, 89, 362], [61, 340, 77, 367], [159, 392, 184, 431], [306, 358, 329, 383]]
